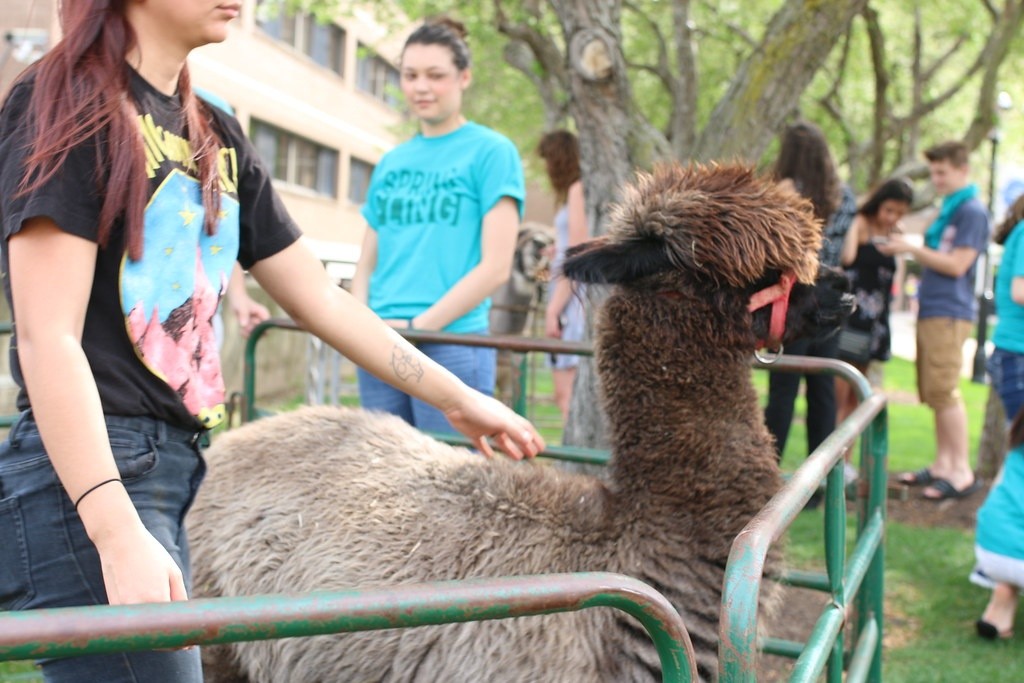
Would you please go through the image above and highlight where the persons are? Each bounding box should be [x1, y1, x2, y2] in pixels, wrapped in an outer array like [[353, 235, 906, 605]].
[[537, 128, 590, 419], [763, 118, 1023, 501], [0, 0, 548, 682], [226, 258, 272, 340], [351, 17, 528, 436], [968, 404, 1024, 640]]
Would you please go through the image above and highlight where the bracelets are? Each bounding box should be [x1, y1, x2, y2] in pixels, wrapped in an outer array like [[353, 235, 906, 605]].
[[408, 317, 412, 329], [74, 479, 122, 510]]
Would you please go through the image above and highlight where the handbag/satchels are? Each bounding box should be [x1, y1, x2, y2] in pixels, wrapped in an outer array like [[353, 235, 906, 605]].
[[834, 329, 871, 371]]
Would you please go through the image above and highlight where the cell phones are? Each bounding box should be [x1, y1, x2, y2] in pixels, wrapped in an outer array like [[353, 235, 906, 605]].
[[872, 236, 892, 246]]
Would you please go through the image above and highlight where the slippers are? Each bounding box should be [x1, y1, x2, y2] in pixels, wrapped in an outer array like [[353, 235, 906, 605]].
[[895, 466, 935, 485], [921, 477, 981, 501]]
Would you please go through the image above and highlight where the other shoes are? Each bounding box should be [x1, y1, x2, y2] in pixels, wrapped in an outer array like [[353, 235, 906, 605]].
[[843, 462, 860, 487]]
